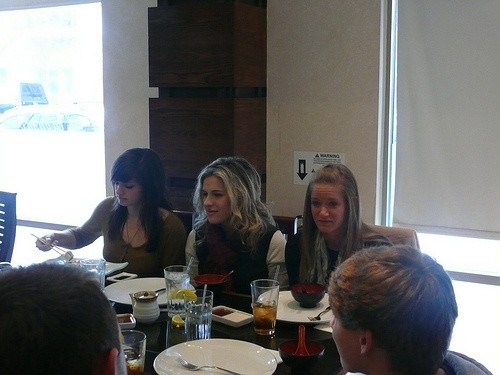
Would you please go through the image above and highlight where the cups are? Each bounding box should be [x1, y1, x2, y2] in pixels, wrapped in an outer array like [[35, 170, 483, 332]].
[[250, 279, 279, 336], [121, 330, 147, 374], [164, 265, 190, 319], [184, 290, 213, 341]]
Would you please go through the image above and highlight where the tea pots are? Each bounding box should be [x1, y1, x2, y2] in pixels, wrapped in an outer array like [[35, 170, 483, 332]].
[[129, 290, 161, 325]]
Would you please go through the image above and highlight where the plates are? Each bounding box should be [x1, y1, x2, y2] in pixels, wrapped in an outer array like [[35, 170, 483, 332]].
[[153, 339, 277, 375], [213, 305, 253, 328], [257, 291, 334, 324], [106, 272, 137, 283], [116, 313, 137, 329], [105, 262, 128, 276], [102, 278, 196, 305]]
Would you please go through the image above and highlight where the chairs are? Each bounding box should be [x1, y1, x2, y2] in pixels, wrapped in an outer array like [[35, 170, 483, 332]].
[[0, 191, 16, 262], [174, 211, 420, 248]]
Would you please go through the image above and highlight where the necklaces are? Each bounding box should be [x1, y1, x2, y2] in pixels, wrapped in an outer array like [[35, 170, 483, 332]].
[[125, 219, 140, 244]]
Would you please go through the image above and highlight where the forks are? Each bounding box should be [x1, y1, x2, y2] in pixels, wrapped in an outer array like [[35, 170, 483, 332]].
[[308, 304, 331, 321], [173, 355, 240, 375]]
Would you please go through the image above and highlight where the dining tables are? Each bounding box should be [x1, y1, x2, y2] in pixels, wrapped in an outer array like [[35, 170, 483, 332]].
[[104, 276, 344, 375]]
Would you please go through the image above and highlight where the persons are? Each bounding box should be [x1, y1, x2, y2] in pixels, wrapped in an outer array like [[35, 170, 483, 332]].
[[186, 157, 290, 293], [328, 243, 493, 375], [0, 263, 127, 375], [285, 164, 393, 293], [35, 147, 187, 276]]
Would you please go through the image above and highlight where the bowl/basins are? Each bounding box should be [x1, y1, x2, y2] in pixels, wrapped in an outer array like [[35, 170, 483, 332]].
[[193, 275, 229, 294], [295, 325, 309, 356], [290, 283, 325, 307], [279, 341, 323, 367]]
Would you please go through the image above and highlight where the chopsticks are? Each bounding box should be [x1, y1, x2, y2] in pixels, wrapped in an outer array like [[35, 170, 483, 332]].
[[32, 233, 66, 256]]
